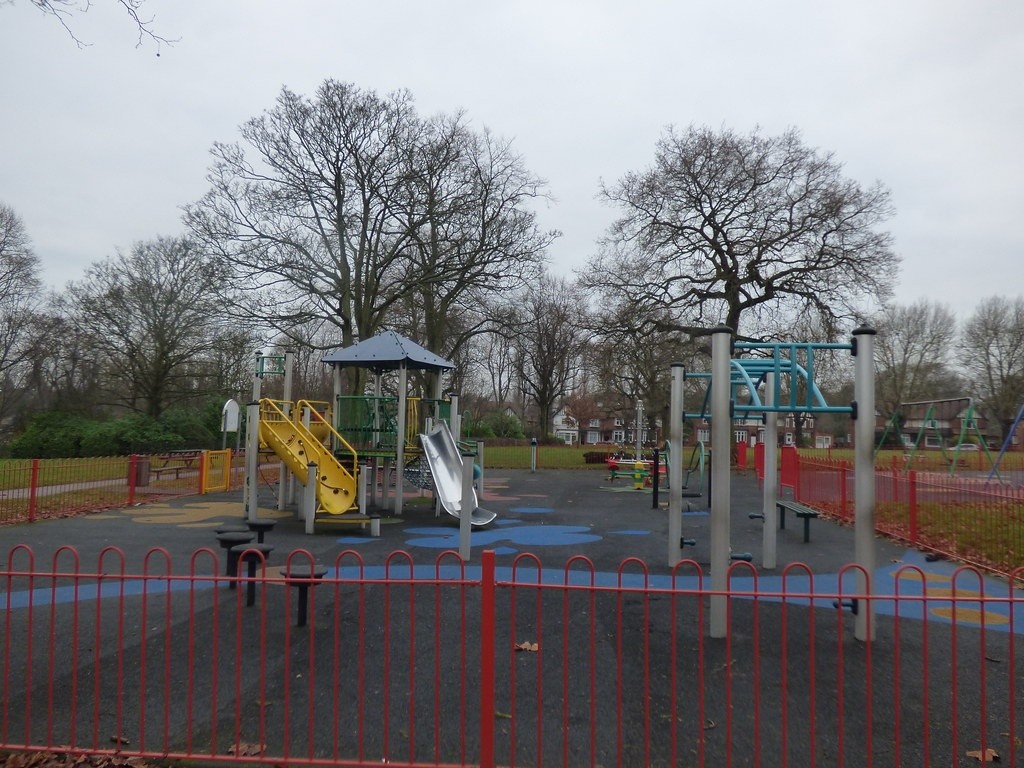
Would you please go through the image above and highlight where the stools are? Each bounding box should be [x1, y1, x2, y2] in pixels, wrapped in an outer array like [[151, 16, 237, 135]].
[[281, 566, 327, 626], [213, 525, 249, 577], [246, 518, 277, 544], [215, 533, 255, 589], [231, 543, 275, 605]]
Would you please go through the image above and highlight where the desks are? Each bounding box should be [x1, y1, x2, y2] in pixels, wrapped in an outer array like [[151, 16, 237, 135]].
[[232, 448, 245, 459], [163, 449, 214, 469]]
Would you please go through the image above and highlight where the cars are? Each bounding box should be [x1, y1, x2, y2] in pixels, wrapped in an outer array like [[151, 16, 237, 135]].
[[946, 443, 979, 451], [593, 441, 657, 447]]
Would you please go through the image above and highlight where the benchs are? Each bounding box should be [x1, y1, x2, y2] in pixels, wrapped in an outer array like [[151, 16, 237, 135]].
[[151, 465, 184, 481], [169, 457, 200, 461], [776, 500, 821, 543], [160, 455, 195, 458], [233, 454, 245, 457]]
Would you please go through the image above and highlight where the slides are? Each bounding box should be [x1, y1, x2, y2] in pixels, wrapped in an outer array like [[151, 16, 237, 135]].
[[258, 398, 358, 515], [418, 418, 497, 527]]
